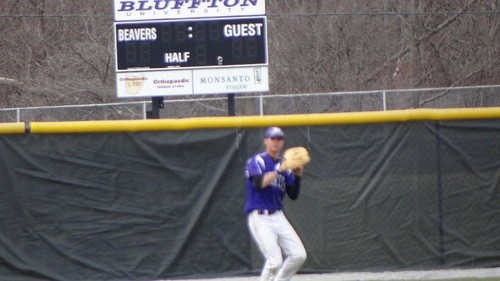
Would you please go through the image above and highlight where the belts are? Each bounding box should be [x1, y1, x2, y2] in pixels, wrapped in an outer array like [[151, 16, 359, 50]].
[[258, 209, 275, 214]]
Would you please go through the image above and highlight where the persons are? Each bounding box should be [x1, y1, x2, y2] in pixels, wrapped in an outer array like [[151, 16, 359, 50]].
[[244, 126, 306, 281]]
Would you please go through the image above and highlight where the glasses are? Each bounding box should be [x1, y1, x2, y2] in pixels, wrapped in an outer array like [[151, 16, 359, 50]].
[[269, 136, 282, 140]]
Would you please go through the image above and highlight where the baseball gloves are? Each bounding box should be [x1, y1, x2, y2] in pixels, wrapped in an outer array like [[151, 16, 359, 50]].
[[280, 146, 311, 172]]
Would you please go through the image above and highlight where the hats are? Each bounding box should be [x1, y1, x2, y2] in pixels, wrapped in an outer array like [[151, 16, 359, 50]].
[[266, 126, 284, 138]]
